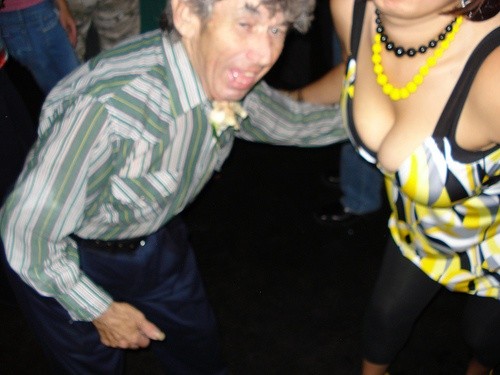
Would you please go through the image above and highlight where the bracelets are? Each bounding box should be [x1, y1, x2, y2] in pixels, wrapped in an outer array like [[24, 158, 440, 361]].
[[296, 87, 304, 103]]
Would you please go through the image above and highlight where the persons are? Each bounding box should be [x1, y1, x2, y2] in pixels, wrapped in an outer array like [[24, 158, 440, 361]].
[[0, 1, 167, 98], [313, 25, 384, 229], [0, 0, 351, 374], [276, 1, 500, 373]]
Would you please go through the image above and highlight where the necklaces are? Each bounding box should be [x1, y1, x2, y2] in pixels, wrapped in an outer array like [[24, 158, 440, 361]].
[[371, 7, 464, 103]]
